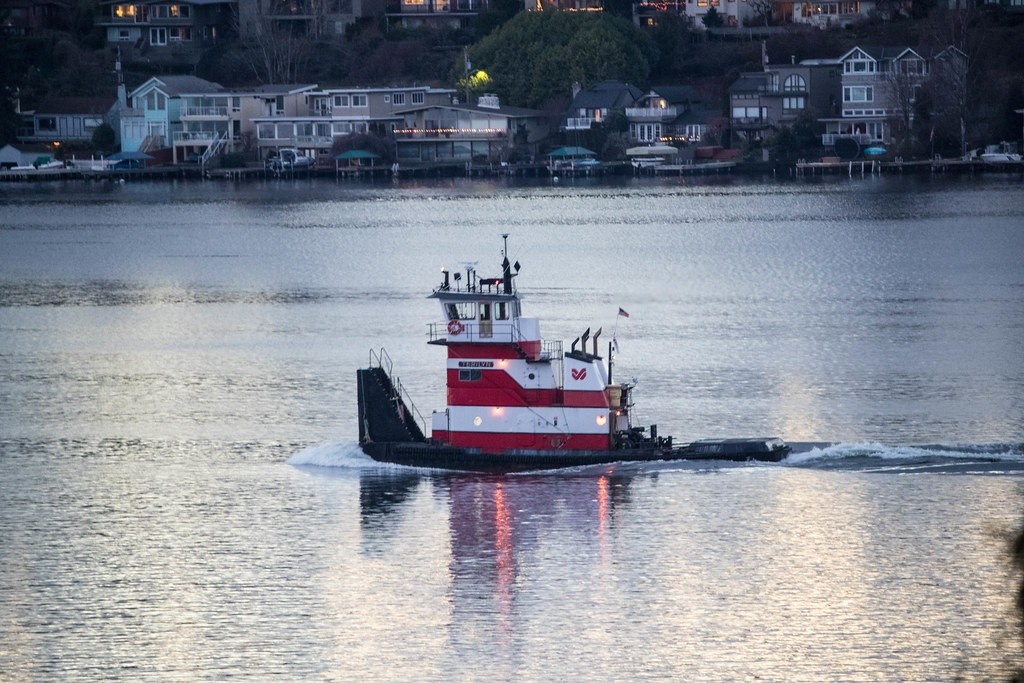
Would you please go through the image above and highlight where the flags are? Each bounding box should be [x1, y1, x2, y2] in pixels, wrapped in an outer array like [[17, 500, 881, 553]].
[[618, 308, 629, 318]]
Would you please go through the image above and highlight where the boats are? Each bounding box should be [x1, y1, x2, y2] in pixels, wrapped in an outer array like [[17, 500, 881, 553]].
[[981, 152, 1023, 163], [354, 231, 789, 465]]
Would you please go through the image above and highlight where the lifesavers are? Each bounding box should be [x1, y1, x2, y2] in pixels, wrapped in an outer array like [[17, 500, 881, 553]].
[[447, 320, 462, 336]]
